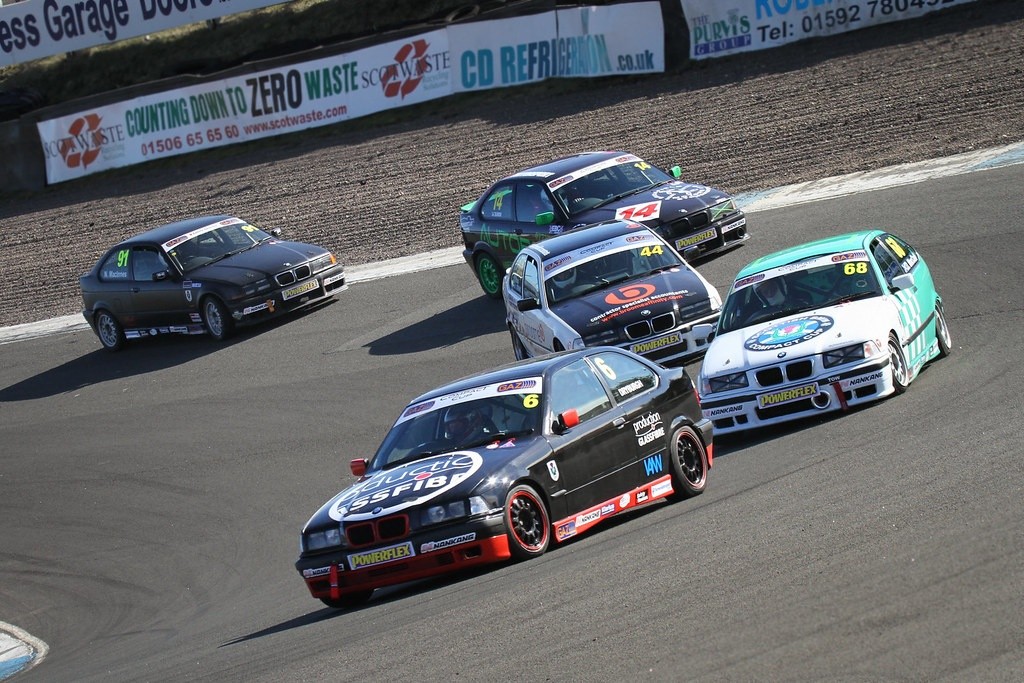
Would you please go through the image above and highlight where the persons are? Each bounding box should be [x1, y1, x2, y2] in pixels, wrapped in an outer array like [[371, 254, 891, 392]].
[[751, 276, 792, 313], [550, 268, 593, 295], [444, 404, 484, 444], [538, 189, 575, 215], [155, 249, 189, 275]]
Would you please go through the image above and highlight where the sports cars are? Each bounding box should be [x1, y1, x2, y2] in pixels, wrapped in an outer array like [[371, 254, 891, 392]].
[[77, 213, 350, 353], [500, 218, 725, 366], [292, 343, 714, 610], [691, 226, 954, 437], [457, 150, 752, 299]]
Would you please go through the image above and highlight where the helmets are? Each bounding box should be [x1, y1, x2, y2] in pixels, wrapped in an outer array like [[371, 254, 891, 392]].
[[552, 267, 576, 288], [158, 252, 169, 268], [540, 187, 569, 211], [444, 403, 482, 449], [753, 276, 788, 308]]
